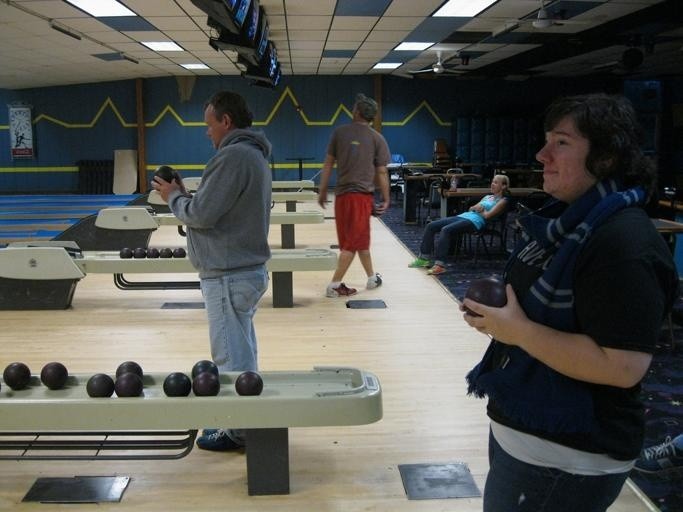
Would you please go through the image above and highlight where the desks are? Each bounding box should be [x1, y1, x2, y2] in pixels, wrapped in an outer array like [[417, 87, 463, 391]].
[[647, 218, 683, 257]]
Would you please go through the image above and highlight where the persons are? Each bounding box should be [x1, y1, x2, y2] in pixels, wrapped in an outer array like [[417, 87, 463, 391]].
[[316, 92, 393, 298], [458, 90, 682, 512], [407, 174, 512, 276], [150, 89, 273, 455]]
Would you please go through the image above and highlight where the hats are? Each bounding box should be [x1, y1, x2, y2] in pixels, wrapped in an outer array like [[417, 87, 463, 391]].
[[355, 91, 378, 120]]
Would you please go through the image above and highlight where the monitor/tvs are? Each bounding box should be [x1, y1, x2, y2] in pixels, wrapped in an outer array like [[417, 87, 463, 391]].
[[191, 0, 282, 90]]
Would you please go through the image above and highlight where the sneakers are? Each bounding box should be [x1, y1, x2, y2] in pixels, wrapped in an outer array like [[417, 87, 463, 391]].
[[325, 282, 356, 298], [407, 258, 431, 268], [427, 265, 447, 274], [366, 271, 381, 289], [196, 429, 245, 452], [631, 440, 682, 474]]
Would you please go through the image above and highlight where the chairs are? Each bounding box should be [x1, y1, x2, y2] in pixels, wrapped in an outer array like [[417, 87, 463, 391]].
[[388, 163, 551, 268]]
